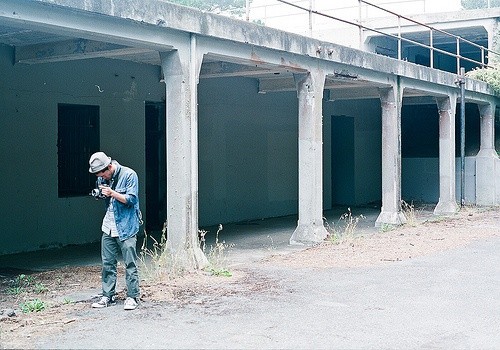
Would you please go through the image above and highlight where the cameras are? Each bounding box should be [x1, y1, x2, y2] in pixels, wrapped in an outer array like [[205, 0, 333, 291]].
[[92, 184, 109, 200]]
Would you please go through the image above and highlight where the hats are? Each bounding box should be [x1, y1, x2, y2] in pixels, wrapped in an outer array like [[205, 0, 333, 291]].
[[89, 152, 112, 173]]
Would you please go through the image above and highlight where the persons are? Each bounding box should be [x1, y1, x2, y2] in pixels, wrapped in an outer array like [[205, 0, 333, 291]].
[[89, 152, 139, 310]]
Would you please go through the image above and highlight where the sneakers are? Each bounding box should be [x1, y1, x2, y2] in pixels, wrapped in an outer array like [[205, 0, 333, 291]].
[[124, 298, 138, 309], [92, 296, 116, 307]]
